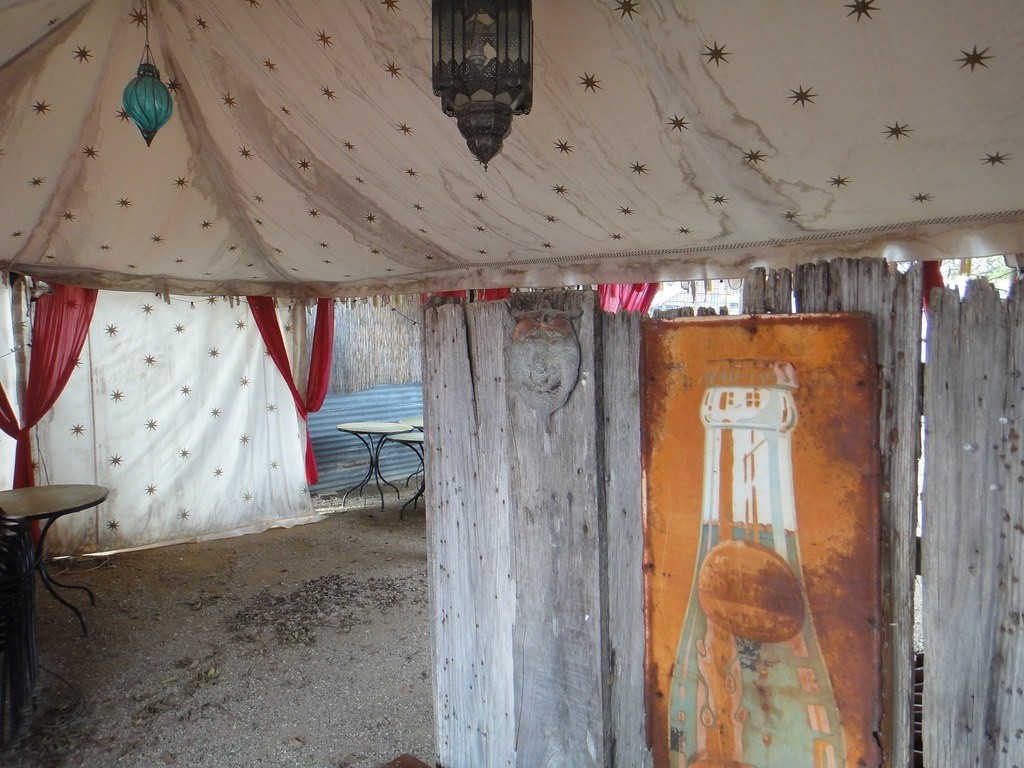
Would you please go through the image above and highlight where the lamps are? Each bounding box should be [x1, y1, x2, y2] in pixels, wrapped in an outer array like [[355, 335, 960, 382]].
[[427, 0, 535, 172], [122, 0, 173, 147]]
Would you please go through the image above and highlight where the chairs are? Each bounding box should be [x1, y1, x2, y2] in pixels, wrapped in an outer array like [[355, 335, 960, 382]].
[[0, 518, 40, 755]]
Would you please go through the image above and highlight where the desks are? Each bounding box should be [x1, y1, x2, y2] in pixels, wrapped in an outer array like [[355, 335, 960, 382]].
[[386, 431, 425, 519], [399, 416, 424, 487], [0, 486, 109, 641], [336, 421, 413, 512]]
